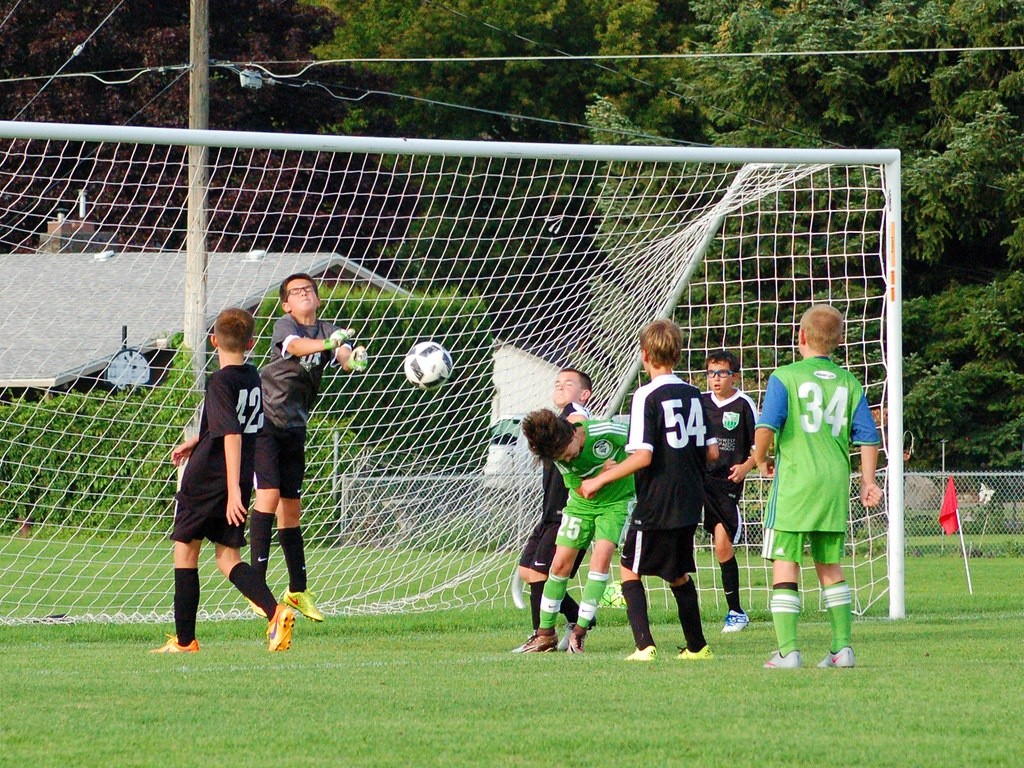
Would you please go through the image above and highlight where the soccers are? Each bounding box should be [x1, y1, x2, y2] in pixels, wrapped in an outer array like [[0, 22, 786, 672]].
[[403, 340, 453, 391]]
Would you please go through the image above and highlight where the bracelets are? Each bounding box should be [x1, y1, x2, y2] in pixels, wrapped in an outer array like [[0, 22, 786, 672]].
[[747, 455, 756, 466]]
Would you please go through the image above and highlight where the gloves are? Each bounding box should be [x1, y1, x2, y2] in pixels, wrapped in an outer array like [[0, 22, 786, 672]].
[[323, 328, 356, 350], [348, 346, 368, 373]]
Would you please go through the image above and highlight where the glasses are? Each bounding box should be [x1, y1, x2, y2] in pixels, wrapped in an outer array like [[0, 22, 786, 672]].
[[706, 369, 734, 378], [286, 285, 317, 302]]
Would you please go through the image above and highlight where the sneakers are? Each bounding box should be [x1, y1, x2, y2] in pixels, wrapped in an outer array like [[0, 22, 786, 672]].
[[674, 645, 715, 660], [558, 617, 596, 651], [763, 651, 803, 669], [242, 595, 268, 618], [283, 586, 325, 623], [566, 630, 585, 654], [267, 605, 295, 651], [623, 644, 657, 662], [510, 631, 558, 652], [150, 634, 199, 653], [817, 647, 855, 669], [721, 610, 750, 633]]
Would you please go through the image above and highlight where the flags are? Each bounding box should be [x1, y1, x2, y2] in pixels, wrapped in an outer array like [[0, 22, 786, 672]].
[[939, 476, 960, 536]]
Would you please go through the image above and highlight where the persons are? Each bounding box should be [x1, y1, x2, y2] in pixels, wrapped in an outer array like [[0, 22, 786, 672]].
[[511, 407, 638, 654], [158, 308, 295, 654], [518, 369, 597, 650], [580, 317, 720, 662], [754, 303, 883, 669], [700, 351, 755, 634], [242, 273, 368, 622]]
